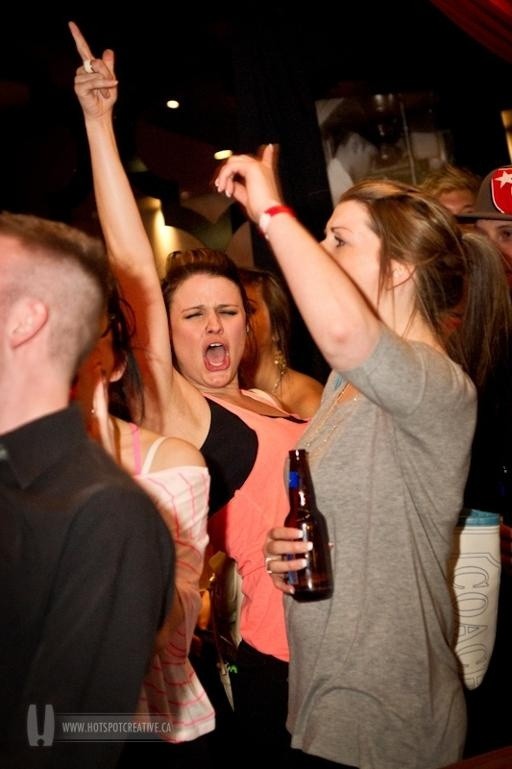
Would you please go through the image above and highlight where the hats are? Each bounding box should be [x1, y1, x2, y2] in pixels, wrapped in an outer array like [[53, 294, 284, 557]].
[[450, 165, 512, 224]]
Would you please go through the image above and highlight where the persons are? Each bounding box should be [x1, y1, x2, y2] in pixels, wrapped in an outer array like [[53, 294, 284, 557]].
[[0, 18, 511, 768]]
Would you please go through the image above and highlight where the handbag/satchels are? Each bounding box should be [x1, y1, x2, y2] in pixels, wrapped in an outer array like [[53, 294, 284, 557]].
[[446, 507, 501, 689]]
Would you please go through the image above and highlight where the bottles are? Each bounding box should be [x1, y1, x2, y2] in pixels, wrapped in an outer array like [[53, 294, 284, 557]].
[[284, 452, 334, 602], [195, 558, 233, 640]]
